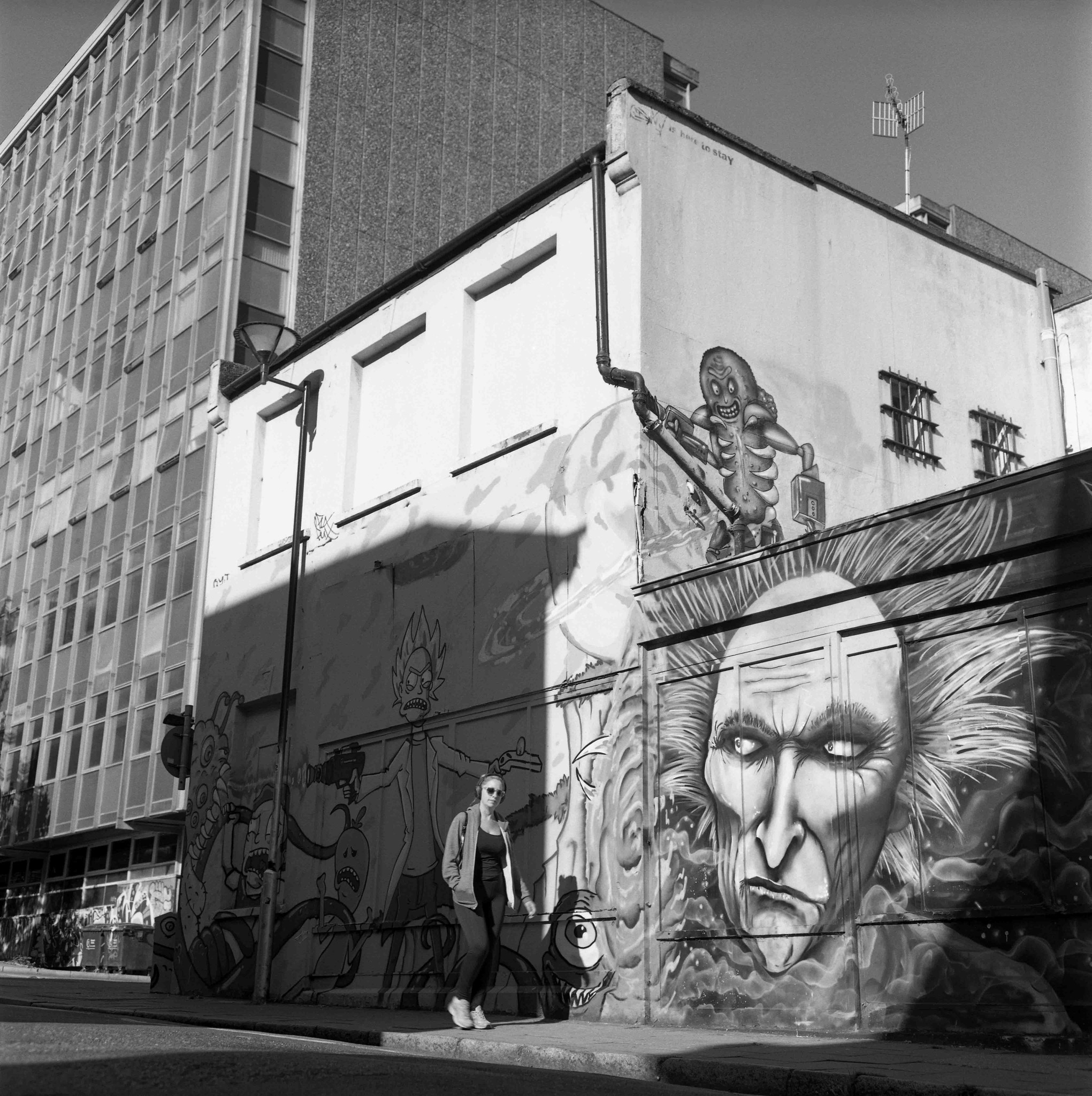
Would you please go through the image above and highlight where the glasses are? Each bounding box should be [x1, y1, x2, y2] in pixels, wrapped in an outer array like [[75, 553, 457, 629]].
[[483, 788, 505, 797]]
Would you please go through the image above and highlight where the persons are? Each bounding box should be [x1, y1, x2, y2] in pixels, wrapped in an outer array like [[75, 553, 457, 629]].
[[442, 773, 537, 1030]]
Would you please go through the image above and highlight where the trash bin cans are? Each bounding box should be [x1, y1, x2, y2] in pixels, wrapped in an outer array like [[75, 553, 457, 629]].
[[80, 923, 111, 973], [103, 922, 155, 976]]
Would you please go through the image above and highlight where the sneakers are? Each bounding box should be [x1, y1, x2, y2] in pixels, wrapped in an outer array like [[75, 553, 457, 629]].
[[470, 1005, 492, 1029], [447, 996, 474, 1029]]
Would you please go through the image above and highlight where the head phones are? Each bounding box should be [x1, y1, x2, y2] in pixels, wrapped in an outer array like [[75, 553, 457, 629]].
[[474, 772, 508, 804]]
